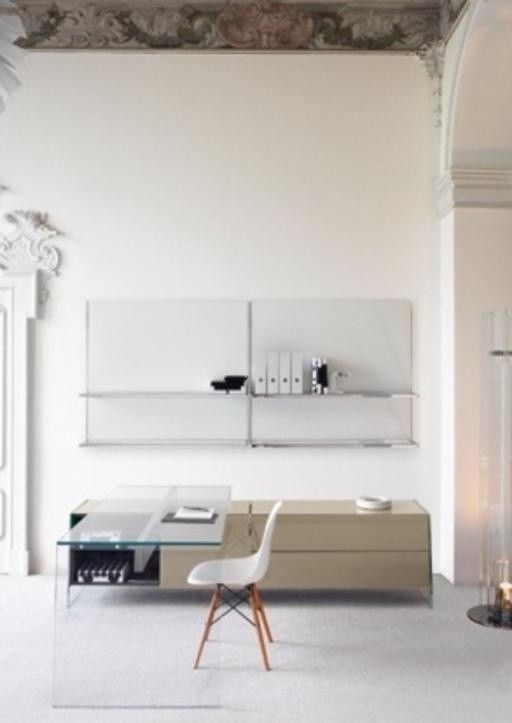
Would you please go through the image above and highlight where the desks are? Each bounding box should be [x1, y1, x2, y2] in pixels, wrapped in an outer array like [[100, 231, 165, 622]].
[[55, 484, 231, 707]]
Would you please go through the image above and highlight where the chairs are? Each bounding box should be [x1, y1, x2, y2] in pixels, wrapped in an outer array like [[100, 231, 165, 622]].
[[188, 499, 282, 671]]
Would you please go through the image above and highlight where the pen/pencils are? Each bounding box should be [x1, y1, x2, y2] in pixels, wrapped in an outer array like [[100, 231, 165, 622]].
[[184, 506, 208, 512]]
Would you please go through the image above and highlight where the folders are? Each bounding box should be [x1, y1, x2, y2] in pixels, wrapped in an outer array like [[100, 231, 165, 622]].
[[255, 350, 303, 394]]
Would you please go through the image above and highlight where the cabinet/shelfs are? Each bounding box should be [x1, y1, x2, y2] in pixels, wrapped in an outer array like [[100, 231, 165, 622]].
[[68, 499, 433, 608], [79, 297, 419, 449]]
